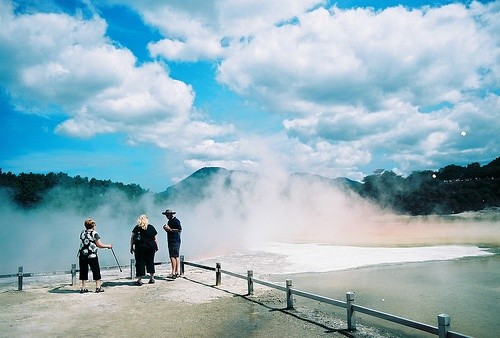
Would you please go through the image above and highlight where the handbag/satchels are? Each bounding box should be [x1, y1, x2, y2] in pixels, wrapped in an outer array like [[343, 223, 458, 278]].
[[132, 231, 140, 244]]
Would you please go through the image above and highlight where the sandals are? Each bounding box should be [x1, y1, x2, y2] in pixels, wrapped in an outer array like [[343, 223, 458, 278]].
[[80, 288, 88, 293], [96, 286, 104, 292]]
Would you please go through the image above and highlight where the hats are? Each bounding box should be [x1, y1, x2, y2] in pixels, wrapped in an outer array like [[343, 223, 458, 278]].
[[162, 210, 176, 215]]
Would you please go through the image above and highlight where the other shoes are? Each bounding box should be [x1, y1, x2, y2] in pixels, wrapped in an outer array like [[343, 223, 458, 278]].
[[167, 273, 176, 279], [135, 281, 142, 285], [148, 279, 155, 284], [176, 272, 180, 277]]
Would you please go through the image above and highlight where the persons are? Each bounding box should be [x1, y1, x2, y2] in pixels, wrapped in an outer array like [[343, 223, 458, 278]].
[[129, 215, 159, 285], [162, 209, 182, 279], [78, 219, 112, 294]]
